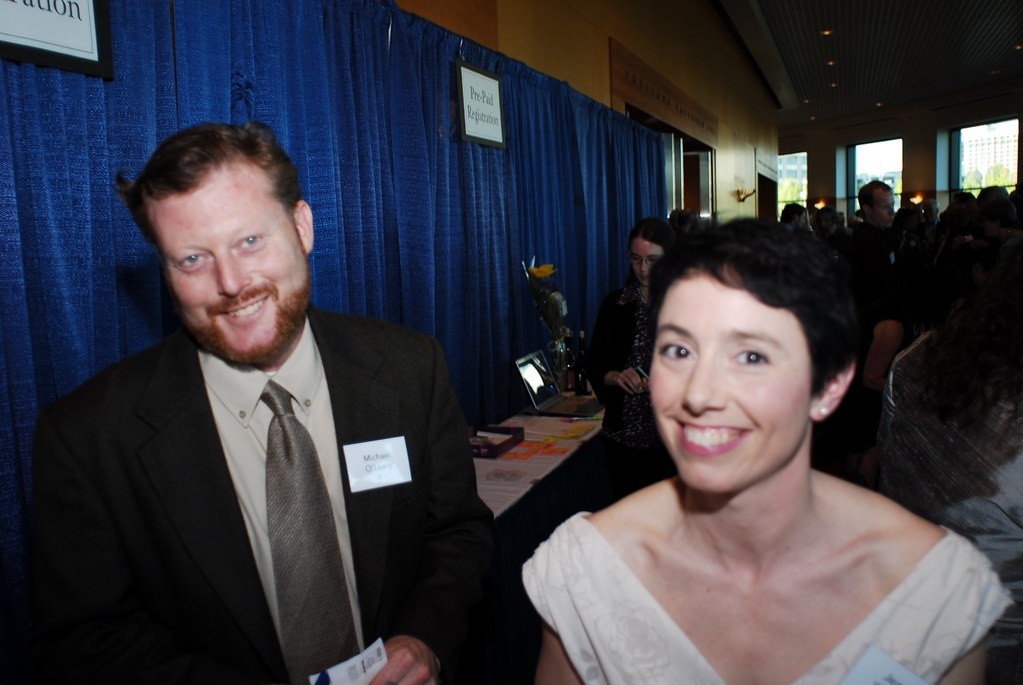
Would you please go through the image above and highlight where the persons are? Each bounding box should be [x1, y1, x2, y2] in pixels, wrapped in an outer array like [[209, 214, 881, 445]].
[[34, 121, 494, 685], [587, 215, 698, 508], [782, 182, 1018, 481], [522, 212, 1013, 684], [877, 242, 1023, 685]]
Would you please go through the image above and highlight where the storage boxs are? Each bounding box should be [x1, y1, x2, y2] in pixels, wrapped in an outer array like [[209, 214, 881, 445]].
[[470, 424, 523, 459]]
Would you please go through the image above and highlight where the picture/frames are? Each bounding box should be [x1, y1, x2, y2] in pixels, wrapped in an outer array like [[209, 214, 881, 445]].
[[0, 0, 116, 83], [453, 58, 508, 148]]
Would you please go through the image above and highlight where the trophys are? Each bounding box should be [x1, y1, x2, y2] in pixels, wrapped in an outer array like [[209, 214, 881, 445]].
[[543, 291, 575, 390]]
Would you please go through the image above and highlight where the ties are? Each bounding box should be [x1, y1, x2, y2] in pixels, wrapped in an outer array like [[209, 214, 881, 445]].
[[260, 380, 362, 685]]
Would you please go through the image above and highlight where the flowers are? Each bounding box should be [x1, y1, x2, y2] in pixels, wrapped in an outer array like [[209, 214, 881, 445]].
[[527, 264, 575, 369]]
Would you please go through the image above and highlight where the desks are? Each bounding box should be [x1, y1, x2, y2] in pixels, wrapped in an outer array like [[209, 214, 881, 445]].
[[474, 389, 604, 520]]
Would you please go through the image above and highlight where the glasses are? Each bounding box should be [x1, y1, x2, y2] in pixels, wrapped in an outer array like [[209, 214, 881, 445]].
[[625, 251, 658, 266]]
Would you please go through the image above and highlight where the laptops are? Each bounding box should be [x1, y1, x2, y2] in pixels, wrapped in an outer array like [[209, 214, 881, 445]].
[[514, 350, 604, 417]]
